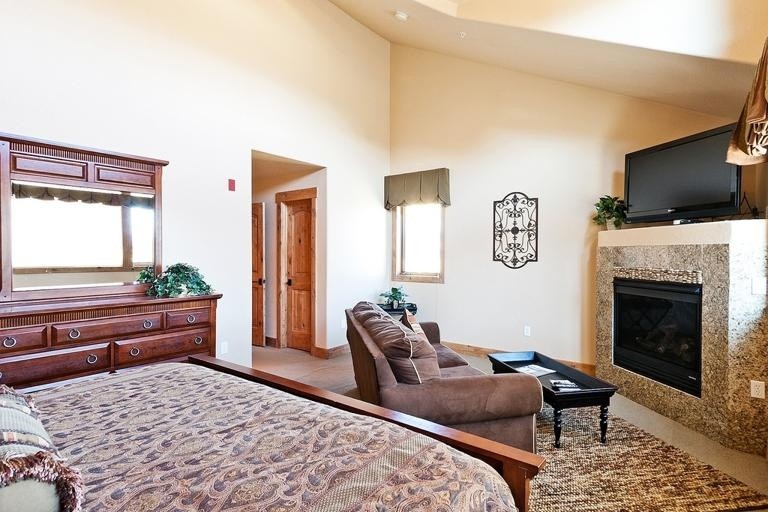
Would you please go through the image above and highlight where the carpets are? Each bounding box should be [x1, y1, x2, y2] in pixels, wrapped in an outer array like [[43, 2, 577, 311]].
[[528, 406, 768, 512]]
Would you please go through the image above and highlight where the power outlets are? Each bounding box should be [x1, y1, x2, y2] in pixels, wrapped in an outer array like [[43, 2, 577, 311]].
[[750, 380, 766, 400]]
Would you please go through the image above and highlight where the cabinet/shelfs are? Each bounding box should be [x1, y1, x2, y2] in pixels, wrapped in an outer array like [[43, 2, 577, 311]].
[[376, 303, 417, 322]]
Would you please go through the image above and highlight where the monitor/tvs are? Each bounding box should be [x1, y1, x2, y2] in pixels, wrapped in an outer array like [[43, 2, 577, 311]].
[[619, 120, 743, 224]]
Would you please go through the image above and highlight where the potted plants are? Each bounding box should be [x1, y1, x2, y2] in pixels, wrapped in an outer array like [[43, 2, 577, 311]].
[[0, 350, 544, 512], [379, 286, 408, 309], [145, 262, 215, 298], [590, 193, 631, 230]]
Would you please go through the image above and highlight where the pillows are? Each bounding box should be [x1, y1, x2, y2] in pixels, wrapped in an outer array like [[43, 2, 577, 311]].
[[0, 384, 86, 512], [362, 313, 442, 384], [352, 301, 396, 323], [400, 311, 430, 344]]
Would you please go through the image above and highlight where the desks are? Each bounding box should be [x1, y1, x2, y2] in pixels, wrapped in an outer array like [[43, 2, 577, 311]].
[[0, 291, 224, 390]]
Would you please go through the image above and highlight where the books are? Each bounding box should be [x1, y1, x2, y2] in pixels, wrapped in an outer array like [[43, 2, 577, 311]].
[[549, 378, 572, 392], [513, 363, 558, 378], [553, 381, 583, 391]]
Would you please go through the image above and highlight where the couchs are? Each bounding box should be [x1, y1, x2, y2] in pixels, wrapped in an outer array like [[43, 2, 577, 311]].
[[345, 309, 544, 455]]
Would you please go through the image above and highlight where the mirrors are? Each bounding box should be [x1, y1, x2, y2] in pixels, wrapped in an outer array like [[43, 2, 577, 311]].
[[0, 132, 170, 303]]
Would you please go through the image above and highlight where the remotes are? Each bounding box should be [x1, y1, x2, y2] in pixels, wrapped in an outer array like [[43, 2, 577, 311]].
[[550, 379, 570, 383], [558, 387, 582, 392], [552, 381, 579, 389]]
